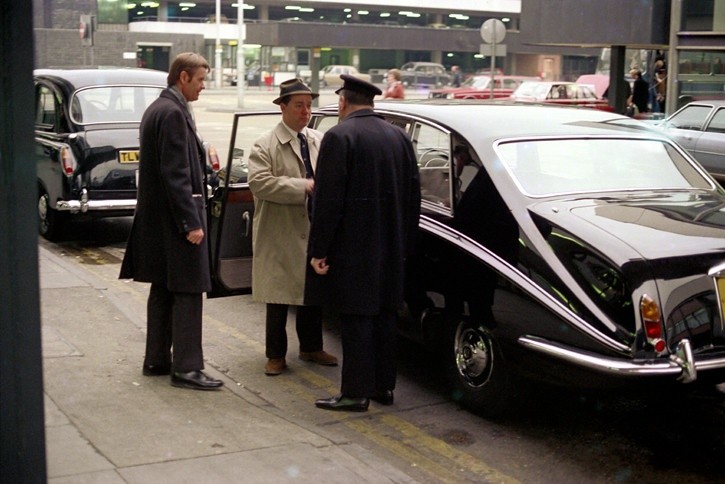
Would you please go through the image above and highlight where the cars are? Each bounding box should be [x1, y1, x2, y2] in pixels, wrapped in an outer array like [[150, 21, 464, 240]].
[[639, 99, 725, 179], [303, 64, 372, 87], [207, 104, 724, 422], [492, 75, 631, 116], [34, 67, 222, 237], [214, 63, 277, 86], [425, 76, 545, 101]]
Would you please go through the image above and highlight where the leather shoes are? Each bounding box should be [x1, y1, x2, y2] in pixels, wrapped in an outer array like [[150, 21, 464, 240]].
[[172, 366, 225, 388], [316, 393, 369, 411], [142, 359, 175, 375], [369, 388, 393, 404]]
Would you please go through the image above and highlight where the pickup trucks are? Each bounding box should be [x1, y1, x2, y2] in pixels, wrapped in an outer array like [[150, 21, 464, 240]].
[[368, 62, 453, 87]]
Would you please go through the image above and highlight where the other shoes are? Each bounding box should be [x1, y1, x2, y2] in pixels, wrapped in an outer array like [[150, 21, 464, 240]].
[[264, 357, 286, 374], [299, 349, 339, 364]]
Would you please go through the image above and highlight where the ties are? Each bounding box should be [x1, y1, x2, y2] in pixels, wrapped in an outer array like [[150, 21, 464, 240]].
[[296, 133, 315, 213]]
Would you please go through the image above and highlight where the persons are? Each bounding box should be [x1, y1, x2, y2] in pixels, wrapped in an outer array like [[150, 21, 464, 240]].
[[117, 53, 226, 390], [621, 63, 668, 115], [310, 74, 422, 412], [451, 65, 461, 87], [246, 79, 339, 374], [385, 68, 406, 100]]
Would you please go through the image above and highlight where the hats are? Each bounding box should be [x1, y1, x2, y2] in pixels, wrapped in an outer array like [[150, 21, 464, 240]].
[[272, 78, 321, 105], [335, 73, 383, 100]]
